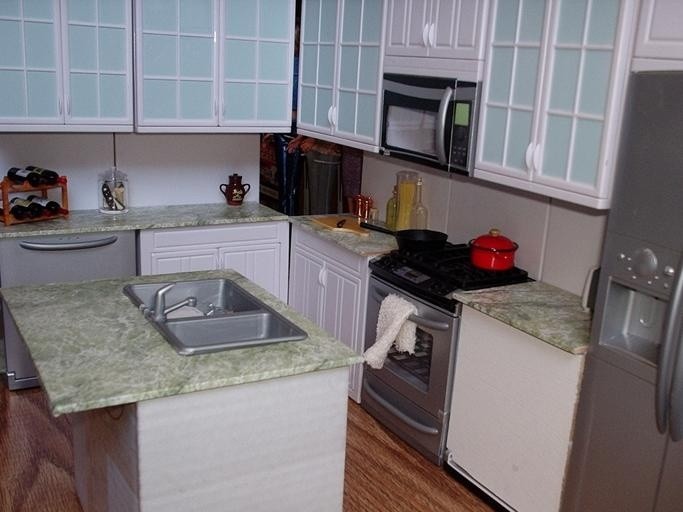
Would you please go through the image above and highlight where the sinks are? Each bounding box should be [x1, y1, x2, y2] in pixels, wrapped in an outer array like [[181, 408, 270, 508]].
[[154, 312, 307, 357], [122, 277, 262, 322]]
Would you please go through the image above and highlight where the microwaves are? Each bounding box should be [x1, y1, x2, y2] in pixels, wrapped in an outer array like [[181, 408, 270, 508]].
[[376, 72, 483, 181]]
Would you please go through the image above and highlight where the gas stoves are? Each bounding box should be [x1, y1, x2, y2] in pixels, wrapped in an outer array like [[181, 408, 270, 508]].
[[368, 244, 529, 313]]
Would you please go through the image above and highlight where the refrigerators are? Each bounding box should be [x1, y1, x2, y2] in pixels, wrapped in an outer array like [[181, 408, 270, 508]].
[[556, 66, 683, 512]]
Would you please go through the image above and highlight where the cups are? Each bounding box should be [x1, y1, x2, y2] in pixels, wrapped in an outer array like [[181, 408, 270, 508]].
[[394, 170, 419, 230]]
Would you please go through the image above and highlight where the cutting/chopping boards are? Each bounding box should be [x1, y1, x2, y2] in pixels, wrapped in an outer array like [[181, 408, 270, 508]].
[[312, 214, 370, 236]]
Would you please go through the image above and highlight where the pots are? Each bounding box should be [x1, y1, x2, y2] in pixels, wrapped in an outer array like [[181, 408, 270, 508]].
[[466, 236, 518, 271], [358, 220, 449, 254]]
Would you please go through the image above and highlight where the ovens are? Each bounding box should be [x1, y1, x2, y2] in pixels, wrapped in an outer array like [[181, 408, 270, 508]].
[[358, 276, 464, 468]]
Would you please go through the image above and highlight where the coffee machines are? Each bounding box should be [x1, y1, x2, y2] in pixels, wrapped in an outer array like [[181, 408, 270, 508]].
[[258, 134, 343, 216]]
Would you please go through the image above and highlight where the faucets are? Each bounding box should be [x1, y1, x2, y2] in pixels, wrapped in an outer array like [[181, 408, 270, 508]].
[[154, 283, 198, 323]]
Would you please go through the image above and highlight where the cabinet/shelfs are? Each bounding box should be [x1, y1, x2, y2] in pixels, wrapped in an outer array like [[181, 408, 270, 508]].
[[282, 224, 372, 406], [384, 0, 485, 59], [298, 0, 389, 155], [0, 0, 135, 136], [135, 224, 288, 301], [468, 3, 633, 209], [129, 1, 296, 136], [627, 2, 683, 77]]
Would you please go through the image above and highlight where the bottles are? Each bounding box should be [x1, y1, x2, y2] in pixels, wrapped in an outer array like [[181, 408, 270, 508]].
[[385, 185, 397, 227], [97, 167, 129, 214], [409, 180, 428, 229], [0, 195, 69, 218], [217, 173, 250, 205], [5, 164, 68, 187], [353, 194, 379, 222]]
